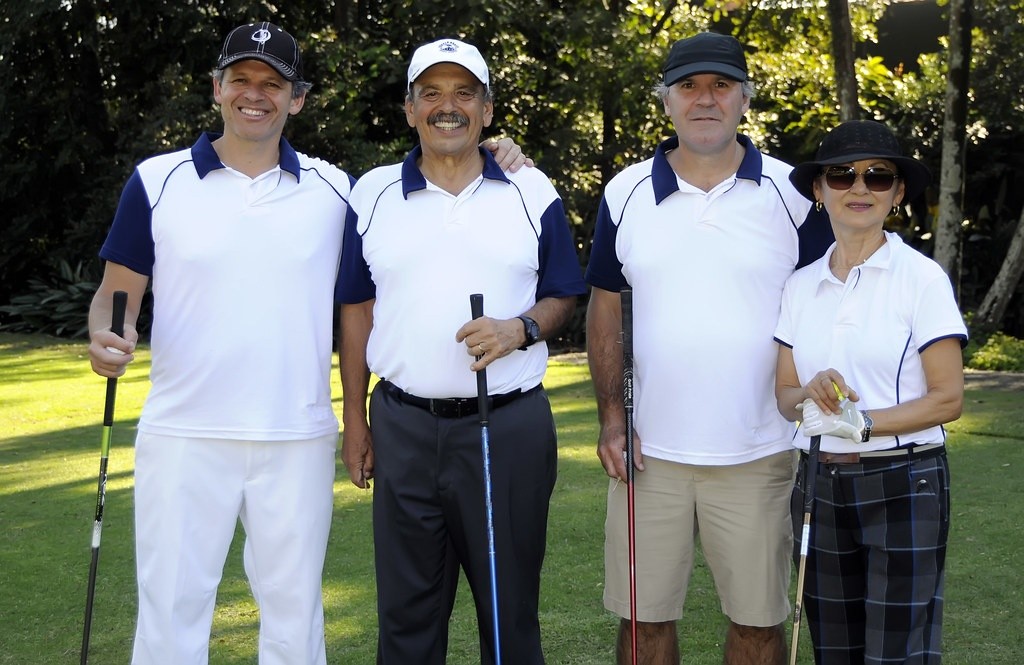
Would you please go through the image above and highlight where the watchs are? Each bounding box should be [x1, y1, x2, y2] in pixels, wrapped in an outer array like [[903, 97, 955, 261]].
[[515, 315, 540, 351], [859, 409, 874, 442]]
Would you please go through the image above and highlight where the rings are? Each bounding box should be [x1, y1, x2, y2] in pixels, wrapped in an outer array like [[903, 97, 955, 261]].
[[478, 345, 487, 354], [819, 375, 829, 384]]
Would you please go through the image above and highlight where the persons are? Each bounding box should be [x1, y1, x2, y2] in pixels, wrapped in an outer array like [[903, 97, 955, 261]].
[[773, 119, 969, 665], [88, 21, 589, 665], [586, 32, 834, 665]]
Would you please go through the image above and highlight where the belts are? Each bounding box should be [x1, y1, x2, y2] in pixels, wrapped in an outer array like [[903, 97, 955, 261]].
[[381, 379, 543, 419], [800, 441, 945, 464]]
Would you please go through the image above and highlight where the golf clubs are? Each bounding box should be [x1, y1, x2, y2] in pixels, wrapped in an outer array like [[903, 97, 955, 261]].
[[469, 292, 503, 665], [789, 434, 822, 665], [79, 289, 129, 665], [618, 285, 638, 665]]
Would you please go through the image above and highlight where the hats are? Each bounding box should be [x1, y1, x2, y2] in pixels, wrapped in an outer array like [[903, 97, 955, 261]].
[[789, 120, 932, 217], [408, 39, 490, 92], [664, 33, 748, 86], [217, 21, 304, 82]]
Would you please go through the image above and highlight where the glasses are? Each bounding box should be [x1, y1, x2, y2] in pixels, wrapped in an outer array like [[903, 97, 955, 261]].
[[819, 166, 903, 192]]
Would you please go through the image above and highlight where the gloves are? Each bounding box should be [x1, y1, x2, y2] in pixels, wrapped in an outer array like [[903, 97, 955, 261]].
[[795, 397, 865, 443]]
